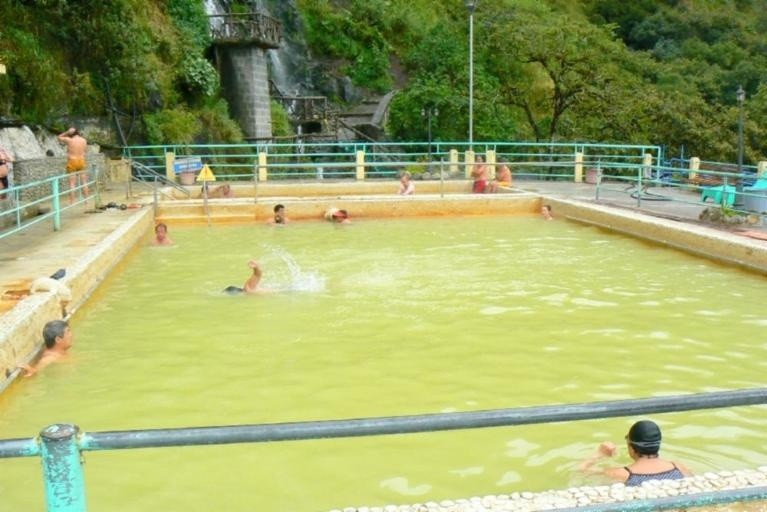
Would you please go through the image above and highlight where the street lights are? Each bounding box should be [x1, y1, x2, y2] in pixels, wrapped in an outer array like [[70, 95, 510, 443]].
[[735, 82, 746, 184], [466, 0, 477, 151]]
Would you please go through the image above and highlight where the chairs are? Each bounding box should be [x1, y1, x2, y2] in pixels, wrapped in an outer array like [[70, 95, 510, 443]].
[[701, 161, 766, 206]]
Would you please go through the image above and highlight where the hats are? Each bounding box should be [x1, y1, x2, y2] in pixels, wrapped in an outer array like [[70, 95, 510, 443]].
[[334, 211, 347, 216], [628, 420, 662, 455]]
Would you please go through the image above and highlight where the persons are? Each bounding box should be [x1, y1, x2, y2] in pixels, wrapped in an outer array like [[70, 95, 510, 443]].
[[332, 209, 351, 224], [397, 171, 414, 195], [198, 185, 209, 199], [14, 318, 73, 379], [0, 145, 16, 201], [222, 260, 262, 295], [541, 204, 552, 222], [57, 127, 89, 208], [215, 183, 234, 199], [265, 204, 291, 224], [147, 222, 174, 246], [573, 418, 691, 486], [469, 155, 488, 192], [488, 159, 512, 193]]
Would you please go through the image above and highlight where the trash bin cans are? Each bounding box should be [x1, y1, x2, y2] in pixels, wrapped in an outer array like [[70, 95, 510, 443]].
[[180, 172, 194, 185], [584, 169, 602, 183]]
